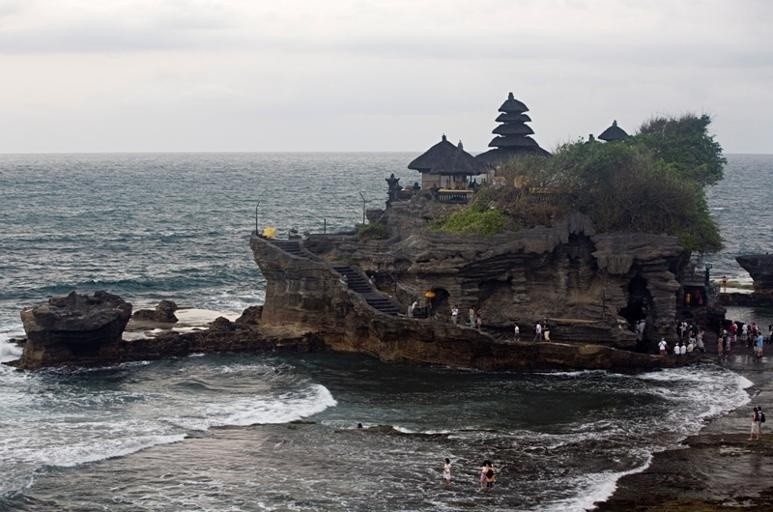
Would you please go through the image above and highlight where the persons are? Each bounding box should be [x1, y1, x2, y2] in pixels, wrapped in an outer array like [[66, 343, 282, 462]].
[[533, 321, 542, 342], [485, 461, 497, 488], [633, 289, 773, 359], [480, 460, 487, 487], [443, 458, 451, 483], [747, 408, 759, 441], [514, 322, 521, 343], [542, 320, 551, 343], [757, 406, 763, 434], [469, 305, 476, 328], [451, 305, 459, 327], [476, 307, 482, 329]]
